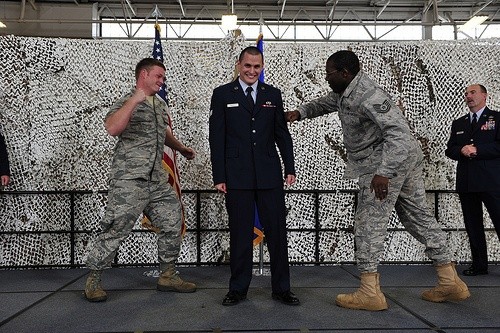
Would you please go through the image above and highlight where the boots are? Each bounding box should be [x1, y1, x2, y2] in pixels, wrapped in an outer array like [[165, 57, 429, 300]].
[[335, 272, 387, 309], [157, 262, 196, 291], [85, 269, 107, 301], [422, 262, 470, 301]]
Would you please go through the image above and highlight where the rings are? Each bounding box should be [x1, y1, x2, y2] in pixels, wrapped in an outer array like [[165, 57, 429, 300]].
[[382, 190, 386, 192]]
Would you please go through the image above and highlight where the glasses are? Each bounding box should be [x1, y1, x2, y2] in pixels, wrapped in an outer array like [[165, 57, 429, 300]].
[[324, 69, 337, 77]]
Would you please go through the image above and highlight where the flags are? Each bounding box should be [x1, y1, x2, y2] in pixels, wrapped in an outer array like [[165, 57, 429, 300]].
[[252, 35, 264, 246], [143, 23, 186, 238]]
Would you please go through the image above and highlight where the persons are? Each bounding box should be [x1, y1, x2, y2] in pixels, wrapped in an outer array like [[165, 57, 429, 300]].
[[285, 50, 470, 310], [85, 59, 195, 301], [210, 47, 300, 306], [445, 84, 500, 275], [0, 133, 10, 185]]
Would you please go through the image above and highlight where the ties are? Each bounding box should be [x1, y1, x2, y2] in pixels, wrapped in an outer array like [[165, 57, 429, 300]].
[[472, 112, 477, 128], [245, 86, 254, 111]]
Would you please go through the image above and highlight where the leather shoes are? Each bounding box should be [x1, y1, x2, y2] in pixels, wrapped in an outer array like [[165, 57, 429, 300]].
[[222, 291, 247, 304], [271, 292, 300, 304], [462, 266, 488, 275]]
